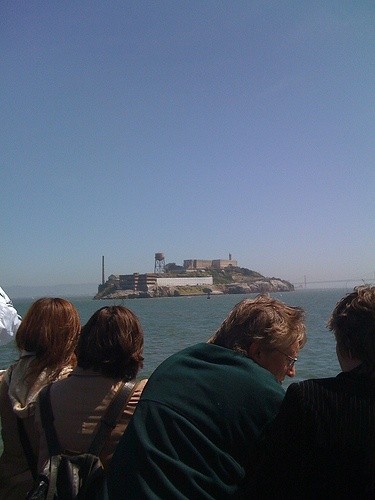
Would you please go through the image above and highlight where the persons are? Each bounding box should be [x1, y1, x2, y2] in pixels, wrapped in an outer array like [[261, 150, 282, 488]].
[[256, 285, 375, 500], [71, 294, 308, 500], [32, 305, 148, 469], [0, 296, 82, 500]]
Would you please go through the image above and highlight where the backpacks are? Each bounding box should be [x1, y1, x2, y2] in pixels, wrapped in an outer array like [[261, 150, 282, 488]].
[[24, 380, 140, 500]]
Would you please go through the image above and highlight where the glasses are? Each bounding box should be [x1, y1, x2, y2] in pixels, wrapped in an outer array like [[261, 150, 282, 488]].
[[274, 348, 297, 367]]
